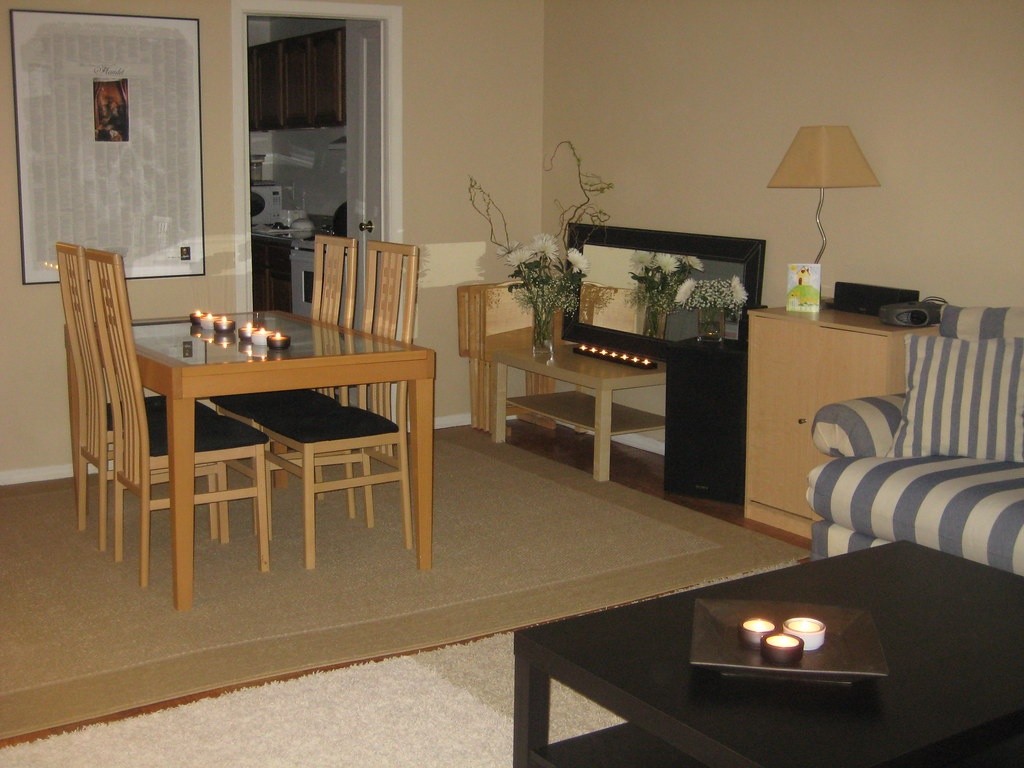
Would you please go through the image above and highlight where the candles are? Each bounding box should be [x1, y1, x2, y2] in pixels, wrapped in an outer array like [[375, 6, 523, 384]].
[[253, 344, 269, 361], [737, 616, 778, 649], [214, 315, 237, 333], [190, 325, 203, 337], [252, 325, 272, 344], [239, 340, 255, 356], [266, 331, 292, 351], [201, 330, 216, 344], [238, 321, 258, 341], [269, 348, 290, 361], [215, 332, 236, 349], [201, 312, 220, 329], [782, 616, 826, 652], [190, 309, 207, 326]]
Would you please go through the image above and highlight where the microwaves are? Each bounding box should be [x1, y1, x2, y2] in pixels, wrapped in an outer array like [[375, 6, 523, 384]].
[[251, 186, 282, 224]]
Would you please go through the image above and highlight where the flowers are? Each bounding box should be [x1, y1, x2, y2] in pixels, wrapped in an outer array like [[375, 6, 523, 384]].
[[465, 136, 616, 312], [676, 279, 746, 311], [627, 253, 700, 308]]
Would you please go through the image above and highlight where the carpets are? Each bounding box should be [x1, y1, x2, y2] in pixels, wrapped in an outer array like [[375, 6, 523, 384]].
[[0, 426, 811, 738], [0, 556, 803, 767]]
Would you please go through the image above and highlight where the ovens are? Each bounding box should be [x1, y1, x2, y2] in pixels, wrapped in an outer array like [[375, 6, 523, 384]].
[[290, 241, 348, 327]]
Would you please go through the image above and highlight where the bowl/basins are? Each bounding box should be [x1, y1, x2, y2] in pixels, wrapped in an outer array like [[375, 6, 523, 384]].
[[290, 218, 315, 229], [281, 210, 307, 228], [272, 222, 284, 229]]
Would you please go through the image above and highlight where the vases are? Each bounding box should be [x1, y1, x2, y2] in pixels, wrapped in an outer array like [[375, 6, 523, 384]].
[[530, 312, 554, 349], [642, 310, 659, 335], [699, 312, 724, 342]]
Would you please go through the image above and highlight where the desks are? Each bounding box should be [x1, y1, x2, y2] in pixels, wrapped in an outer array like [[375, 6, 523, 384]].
[[485, 326, 666, 484], [455, 281, 558, 432], [63, 311, 439, 610]]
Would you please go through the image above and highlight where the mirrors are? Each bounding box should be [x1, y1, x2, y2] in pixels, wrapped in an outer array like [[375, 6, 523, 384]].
[[559, 220, 765, 360]]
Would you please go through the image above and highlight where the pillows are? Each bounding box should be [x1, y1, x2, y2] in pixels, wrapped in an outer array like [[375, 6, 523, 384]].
[[885, 327, 1021, 462]]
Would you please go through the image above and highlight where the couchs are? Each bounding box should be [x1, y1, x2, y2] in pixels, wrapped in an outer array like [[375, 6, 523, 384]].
[[810, 301, 1024, 574]]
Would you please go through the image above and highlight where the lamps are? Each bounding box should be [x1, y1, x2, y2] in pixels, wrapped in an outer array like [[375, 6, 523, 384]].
[[767, 124, 881, 264]]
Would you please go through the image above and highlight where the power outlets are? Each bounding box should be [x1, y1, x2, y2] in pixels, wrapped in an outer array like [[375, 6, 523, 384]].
[[182, 340, 194, 359], [180, 246, 191, 261]]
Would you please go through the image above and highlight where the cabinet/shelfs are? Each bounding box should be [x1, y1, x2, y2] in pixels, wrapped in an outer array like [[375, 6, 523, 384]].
[[740, 306, 939, 535], [253, 245, 274, 310], [252, 238, 293, 311], [248, 26, 347, 129]]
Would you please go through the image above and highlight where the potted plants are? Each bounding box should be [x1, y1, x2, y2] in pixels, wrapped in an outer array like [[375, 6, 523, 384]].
[[758, 633, 804, 666]]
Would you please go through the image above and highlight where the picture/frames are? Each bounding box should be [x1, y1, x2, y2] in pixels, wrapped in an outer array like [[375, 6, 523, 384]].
[[9, 8, 206, 285]]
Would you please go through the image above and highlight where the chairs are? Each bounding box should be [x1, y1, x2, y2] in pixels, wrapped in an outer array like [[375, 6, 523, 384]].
[[211, 233, 357, 518], [54, 237, 218, 549], [265, 242, 420, 571], [83, 247, 271, 589]]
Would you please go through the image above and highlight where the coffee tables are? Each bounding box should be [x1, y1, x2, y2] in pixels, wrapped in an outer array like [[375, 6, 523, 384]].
[[512, 542, 1024, 767]]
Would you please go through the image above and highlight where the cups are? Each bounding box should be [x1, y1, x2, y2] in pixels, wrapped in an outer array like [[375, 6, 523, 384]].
[[250, 162, 262, 180], [250, 154, 266, 162]]
